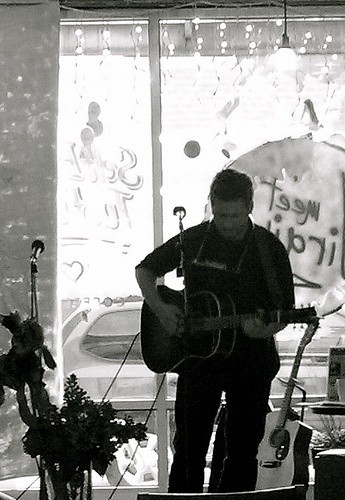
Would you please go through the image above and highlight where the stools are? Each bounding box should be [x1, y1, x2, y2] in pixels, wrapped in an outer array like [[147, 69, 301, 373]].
[[313, 448, 345, 500]]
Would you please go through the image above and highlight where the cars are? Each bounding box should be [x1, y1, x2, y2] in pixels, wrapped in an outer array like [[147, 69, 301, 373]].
[[63, 299, 179, 406]]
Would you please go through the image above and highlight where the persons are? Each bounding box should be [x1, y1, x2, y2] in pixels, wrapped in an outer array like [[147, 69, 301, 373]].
[[134, 170, 295, 494]]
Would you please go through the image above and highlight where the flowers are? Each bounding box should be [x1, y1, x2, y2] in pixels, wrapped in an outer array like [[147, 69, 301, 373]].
[[310, 429, 345, 448]]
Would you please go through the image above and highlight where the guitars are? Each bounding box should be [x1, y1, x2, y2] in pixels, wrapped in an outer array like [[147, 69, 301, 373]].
[[140, 286, 317, 372], [258, 317, 316, 489]]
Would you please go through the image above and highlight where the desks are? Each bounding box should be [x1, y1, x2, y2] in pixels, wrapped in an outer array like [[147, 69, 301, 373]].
[[309, 406, 345, 435]]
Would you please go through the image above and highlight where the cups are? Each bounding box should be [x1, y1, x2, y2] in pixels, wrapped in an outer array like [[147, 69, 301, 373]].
[[336, 379, 345, 402]]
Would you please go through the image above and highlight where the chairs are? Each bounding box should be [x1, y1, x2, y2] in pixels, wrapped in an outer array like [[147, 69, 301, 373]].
[[137, 483, 306, 500]]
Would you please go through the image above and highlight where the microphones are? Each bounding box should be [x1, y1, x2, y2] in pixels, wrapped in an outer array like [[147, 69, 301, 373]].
[[30, 240, 45, 262], [173, 206, 186, 231]]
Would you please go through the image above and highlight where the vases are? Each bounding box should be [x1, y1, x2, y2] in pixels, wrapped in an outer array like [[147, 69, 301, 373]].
[[311, 449, 326, 469]]
